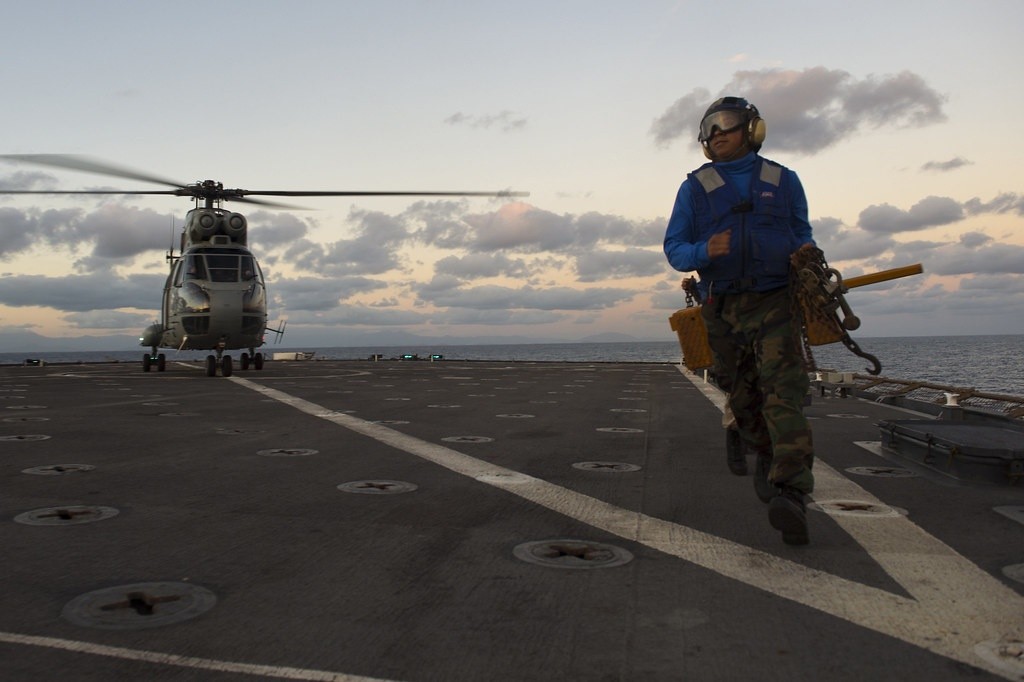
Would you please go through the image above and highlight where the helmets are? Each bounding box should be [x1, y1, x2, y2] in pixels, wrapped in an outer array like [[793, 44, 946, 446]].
[[698, 96, 765, 163]]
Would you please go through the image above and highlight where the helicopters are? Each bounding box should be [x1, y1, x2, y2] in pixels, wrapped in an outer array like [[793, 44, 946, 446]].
[[1, 151, 530, 380]]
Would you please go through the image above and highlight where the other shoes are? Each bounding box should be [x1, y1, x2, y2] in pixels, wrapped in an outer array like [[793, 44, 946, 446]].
[[725, 427, 749, 476], [754, 451, 772, 505], [769, 496, 809, 545]]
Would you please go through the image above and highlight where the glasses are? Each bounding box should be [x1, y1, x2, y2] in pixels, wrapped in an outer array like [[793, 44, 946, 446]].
[[700, 109, 745, 141]]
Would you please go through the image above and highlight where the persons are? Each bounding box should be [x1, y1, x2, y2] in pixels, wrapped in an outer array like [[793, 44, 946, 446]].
[[662, 95, 828, 548]]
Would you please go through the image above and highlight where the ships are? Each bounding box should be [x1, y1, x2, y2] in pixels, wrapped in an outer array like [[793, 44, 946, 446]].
[[1, 355, 1023, 682]]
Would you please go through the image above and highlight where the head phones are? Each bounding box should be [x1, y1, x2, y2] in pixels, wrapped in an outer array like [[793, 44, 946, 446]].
[[698, 98, 766, 159]]
